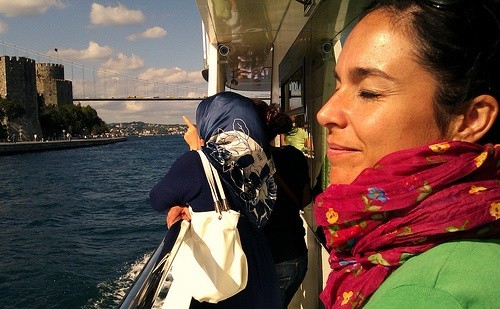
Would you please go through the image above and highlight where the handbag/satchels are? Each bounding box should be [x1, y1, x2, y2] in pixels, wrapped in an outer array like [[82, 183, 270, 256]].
[[170, 149, 249, 304]]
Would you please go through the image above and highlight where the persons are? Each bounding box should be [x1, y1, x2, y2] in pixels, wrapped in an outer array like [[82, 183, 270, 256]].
[[315, 0, 499, 309], [149, 91, 312, 309]]
